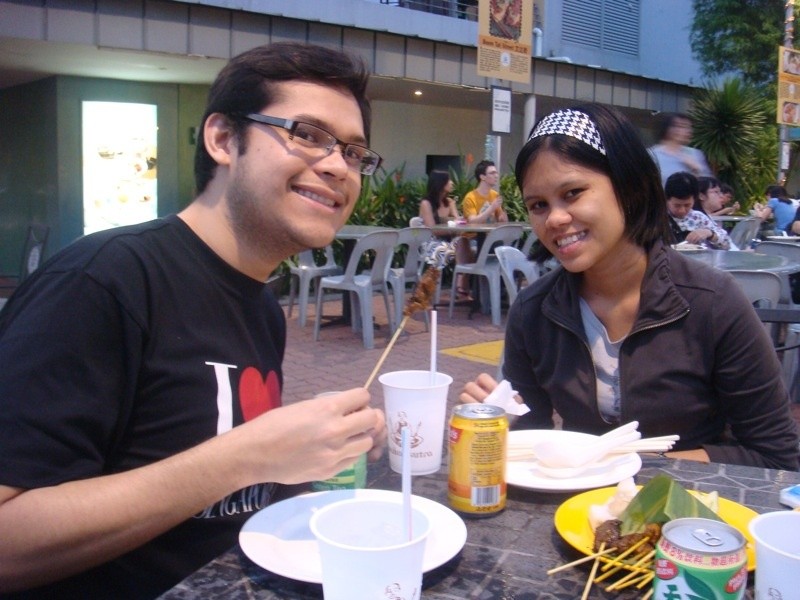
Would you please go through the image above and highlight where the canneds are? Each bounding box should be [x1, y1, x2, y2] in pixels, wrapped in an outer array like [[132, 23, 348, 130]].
[[652, 517, 748, 600], [311, 390, 367, 492], [448, 403, 508, 517], [751, 238, 761, 249]]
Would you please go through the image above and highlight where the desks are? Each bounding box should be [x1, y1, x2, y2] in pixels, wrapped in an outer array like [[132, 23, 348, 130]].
[[676, 249, 800, 306], [428, 222, 532, 319], [154, 456, 800, 600], [319, 224, 396, 329]]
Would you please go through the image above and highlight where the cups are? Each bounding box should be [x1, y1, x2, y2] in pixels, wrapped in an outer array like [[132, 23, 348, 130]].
[[311, 498, 430, 598], [748, 510, 799, 599], [377, 369, 454, 476], [312, 390, 366, 491]]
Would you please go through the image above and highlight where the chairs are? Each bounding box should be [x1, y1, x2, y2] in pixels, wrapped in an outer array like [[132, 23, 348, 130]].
[[0, 224, 50, 310], [283, 216, 800, 398]]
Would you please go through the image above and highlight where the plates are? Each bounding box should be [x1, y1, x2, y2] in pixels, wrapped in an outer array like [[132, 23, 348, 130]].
[[505, 429, 642, 493], [238, 488, 470, 584], [766, 236, 800, 241], [675, 246, 708, 251], [554, 486, 760, 571]]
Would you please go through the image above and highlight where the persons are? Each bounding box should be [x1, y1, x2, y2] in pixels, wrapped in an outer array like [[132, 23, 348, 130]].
[[418, 168, 479, 302], [461, 160, 513, 263], [0, 42, 388, 600], [459, 103, 800, 474], [642, 109, 800, 252]]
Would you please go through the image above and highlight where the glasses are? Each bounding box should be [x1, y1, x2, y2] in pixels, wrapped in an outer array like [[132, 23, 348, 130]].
[[245, 113, 382, 175]]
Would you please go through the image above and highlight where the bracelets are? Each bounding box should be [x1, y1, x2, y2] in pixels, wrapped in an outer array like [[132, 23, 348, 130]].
[[707, 230, 715, 240]]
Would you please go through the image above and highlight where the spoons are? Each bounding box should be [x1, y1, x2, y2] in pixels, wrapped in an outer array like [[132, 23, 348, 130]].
[[506, 419, 679, 479]]
